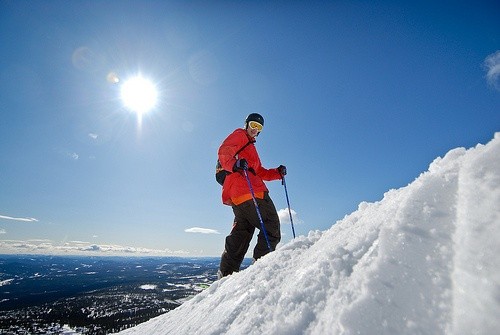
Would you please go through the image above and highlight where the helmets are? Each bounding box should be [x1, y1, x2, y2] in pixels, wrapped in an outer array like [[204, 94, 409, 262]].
[[246, 113, 264, 126]]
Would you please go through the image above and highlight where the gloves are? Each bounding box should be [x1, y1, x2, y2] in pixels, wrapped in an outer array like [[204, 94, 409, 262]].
[[278, 165, 286, 176], [234, 159, 248, 172]]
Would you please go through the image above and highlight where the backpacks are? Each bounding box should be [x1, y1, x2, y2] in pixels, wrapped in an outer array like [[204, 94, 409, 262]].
[[215, 159, 231, 186]]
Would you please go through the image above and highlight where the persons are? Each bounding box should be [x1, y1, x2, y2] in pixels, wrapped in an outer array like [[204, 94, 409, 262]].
[[217, 112, 287, 278]]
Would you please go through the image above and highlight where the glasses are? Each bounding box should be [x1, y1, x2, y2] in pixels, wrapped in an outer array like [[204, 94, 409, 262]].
[[249, 121, 263, 131]]
